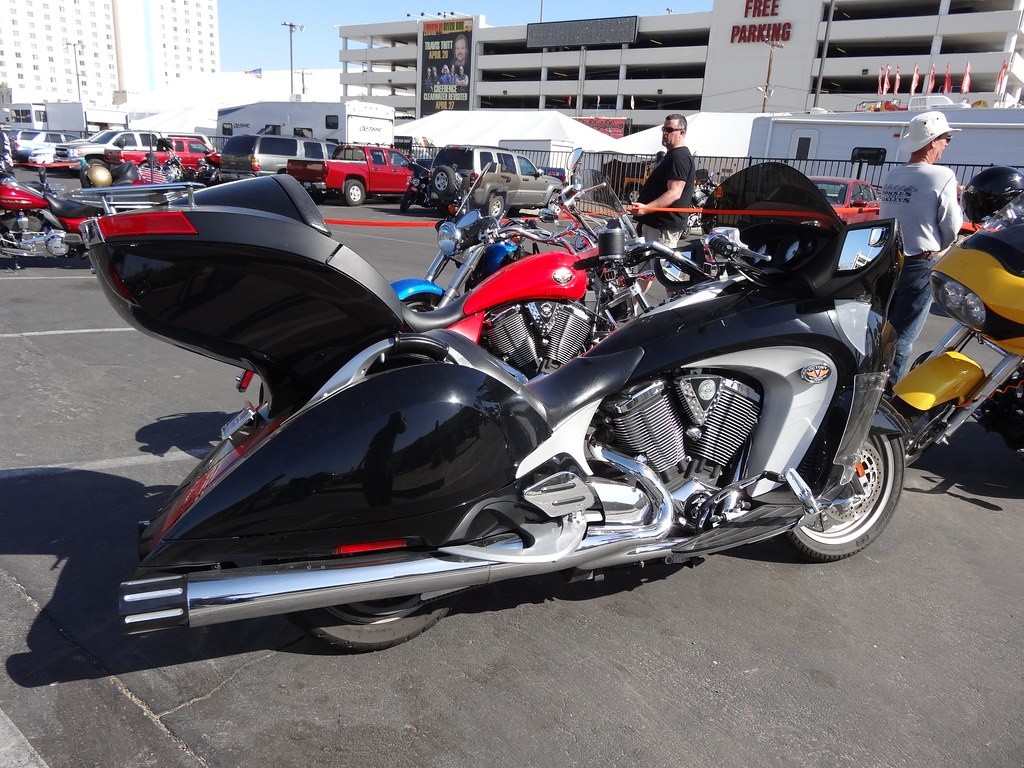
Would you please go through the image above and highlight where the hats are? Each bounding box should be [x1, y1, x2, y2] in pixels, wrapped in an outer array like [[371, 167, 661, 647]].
[[901, 111, 962, 153]]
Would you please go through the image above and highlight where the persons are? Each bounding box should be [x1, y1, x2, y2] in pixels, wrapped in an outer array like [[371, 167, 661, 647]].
[[627, 114, 696, 274], [426, 35, 469, 86], [879, 113, 963, 402]]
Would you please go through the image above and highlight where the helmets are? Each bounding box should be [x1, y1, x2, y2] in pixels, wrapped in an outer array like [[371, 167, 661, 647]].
[[964, 167, 1024, 224], [86, 165, 112, 187]]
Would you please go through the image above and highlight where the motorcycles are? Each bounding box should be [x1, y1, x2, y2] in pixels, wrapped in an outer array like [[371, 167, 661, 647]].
[[889, 202, 1024, 467], [0, 157, 135, 274], [164, 147, 221, 187], [400, 161, 438, 213], [80, 136, 909, 651]]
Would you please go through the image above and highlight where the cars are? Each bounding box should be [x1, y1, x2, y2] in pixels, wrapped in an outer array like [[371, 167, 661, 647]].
[[806, 175, 881, 223], [28, 139, 89, 167]]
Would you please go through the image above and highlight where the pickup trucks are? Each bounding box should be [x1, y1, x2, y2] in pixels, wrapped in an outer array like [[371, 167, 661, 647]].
[[288, 144, 415, 207], [53, 129, 214, 176], [104, 136, 221, 172]]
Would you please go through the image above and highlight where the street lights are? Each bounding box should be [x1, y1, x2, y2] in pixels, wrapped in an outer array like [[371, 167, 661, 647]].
[[281, 22, 304, 93], [66, 42, 86, 101]]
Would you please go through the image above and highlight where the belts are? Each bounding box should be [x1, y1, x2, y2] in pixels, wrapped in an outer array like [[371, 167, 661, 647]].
[[914, 252, 929, 260]]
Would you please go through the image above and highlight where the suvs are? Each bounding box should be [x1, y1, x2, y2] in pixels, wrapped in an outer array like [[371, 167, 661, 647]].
[[429, 145, 566, 216], [8, 131, 81, 161], [217, 133, 339, 183]]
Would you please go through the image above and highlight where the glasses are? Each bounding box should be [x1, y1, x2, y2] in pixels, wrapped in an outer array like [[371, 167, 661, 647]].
[[946, 135, 953, 143], [663, 126, 682, 133]]
